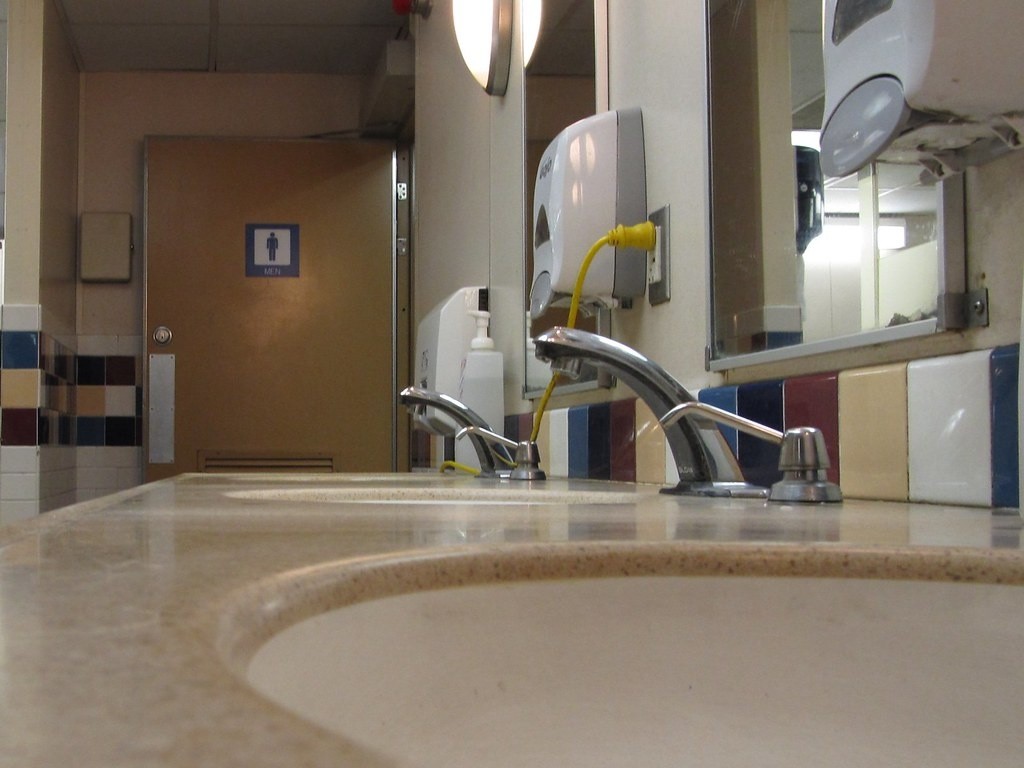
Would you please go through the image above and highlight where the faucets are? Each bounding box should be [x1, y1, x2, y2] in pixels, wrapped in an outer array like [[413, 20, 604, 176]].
[[402, 388, 519, 480], [532, 327, 771, 500]]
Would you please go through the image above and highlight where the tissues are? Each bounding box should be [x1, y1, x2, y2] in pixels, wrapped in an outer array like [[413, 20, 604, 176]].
[[406, 283, 489, 441]]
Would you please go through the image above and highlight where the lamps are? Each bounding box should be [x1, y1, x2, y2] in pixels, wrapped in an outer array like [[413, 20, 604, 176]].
[[452, 0, 513, 96], [521, 0, 546, 72]]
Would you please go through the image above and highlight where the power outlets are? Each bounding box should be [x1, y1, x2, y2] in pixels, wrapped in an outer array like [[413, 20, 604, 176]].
[[648, 203, 671, 308]]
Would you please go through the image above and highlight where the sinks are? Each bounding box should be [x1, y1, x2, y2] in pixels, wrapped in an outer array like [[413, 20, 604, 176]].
[[211, 532, 1023, 768], [222, 478, 652, 507]]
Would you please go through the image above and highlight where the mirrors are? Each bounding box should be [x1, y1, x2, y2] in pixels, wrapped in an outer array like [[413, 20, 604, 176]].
[[522, 0, 617, 402], [705, 0, 967, 374]]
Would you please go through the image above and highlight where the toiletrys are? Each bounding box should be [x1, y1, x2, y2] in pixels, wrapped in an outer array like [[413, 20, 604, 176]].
[[527, 104, 650, 322], [818, 0, 1023, 191], [453, 308, 509, 475]]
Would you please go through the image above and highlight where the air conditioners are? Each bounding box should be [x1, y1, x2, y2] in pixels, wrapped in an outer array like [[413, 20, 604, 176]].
[[358, 38, 415, 135]]
